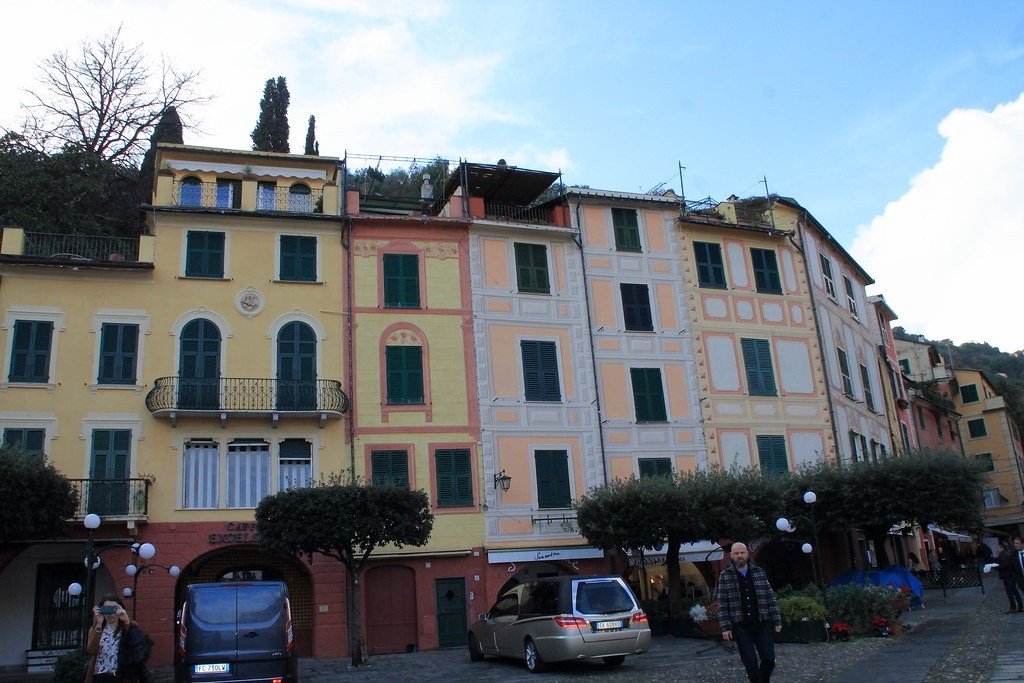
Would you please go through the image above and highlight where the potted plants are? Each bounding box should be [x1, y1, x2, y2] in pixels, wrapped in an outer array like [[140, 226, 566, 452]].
[[897, 397, 908, 407]]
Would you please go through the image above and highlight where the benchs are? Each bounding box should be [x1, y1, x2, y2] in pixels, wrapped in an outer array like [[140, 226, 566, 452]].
[[911, 566, 986, 596]]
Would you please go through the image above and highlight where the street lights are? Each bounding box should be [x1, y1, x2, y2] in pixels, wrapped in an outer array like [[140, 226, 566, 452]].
[[774, 491, 835, 646], [68, 508, 183, 683]]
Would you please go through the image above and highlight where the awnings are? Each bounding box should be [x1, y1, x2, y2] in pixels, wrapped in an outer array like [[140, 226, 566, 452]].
[[618, 540, 723, 567], [932, 529, 972, 543]]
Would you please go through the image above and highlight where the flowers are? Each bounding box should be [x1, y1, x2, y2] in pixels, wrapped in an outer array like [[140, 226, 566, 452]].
[[691, 580, 913, 632]]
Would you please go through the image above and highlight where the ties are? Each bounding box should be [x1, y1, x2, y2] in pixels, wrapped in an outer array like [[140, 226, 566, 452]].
[[1021, 553, 1024, 568]]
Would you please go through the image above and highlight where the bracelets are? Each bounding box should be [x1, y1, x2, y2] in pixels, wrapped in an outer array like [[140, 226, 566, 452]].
[[125, 619, 131, 625], [94, 626, 103, 633]]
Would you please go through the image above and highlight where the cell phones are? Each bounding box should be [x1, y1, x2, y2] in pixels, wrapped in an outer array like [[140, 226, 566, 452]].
[[99, 606, 117, 614]]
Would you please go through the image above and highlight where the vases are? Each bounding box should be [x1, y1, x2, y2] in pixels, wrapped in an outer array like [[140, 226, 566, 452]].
[[109, 253, 123, 262], [891, 621, 903, 634], [890, 597, 910, 610], [700, 619, 723, 636]]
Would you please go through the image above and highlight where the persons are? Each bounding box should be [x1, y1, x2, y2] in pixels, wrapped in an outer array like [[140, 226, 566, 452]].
[[86, 593, 154, 683], [908, 537, 1024, 614], [717, 542, 783, 683]]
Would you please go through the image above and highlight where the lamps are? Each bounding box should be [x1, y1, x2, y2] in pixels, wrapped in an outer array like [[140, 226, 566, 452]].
[[494, 468, 511, 491]]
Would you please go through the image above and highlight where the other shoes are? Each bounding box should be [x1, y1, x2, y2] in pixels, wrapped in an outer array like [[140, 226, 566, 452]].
[[1018, 607, 1024, 612], [1005, 608, 1017, 614]]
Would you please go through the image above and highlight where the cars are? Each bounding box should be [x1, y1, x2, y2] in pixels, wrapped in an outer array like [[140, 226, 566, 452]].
[[467, 576, 653, 669], [176, 580, 298, 683]]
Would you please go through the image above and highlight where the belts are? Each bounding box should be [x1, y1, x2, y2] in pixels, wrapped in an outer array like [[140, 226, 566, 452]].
[[96, 672, 117, 676]]
